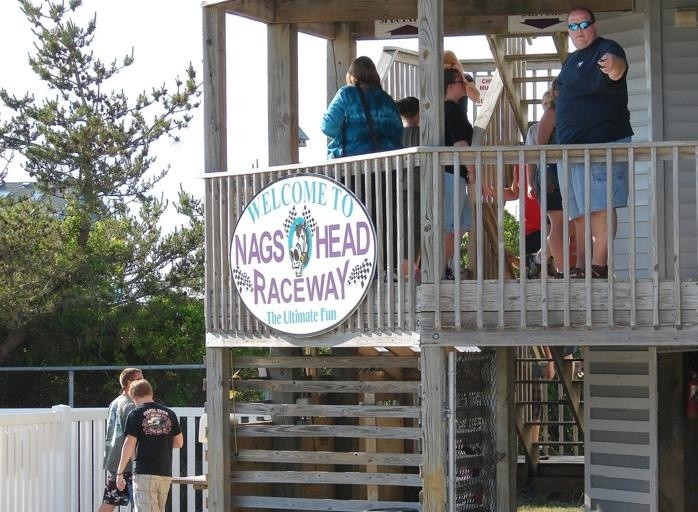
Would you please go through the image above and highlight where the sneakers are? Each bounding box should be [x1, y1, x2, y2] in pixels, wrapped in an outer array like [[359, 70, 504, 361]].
[[381, 267, 421, 285], [445, 262, 468, 281], [547, 257, 608, 279], [538, 449, 549, 459], [524, 252, 540, 278]]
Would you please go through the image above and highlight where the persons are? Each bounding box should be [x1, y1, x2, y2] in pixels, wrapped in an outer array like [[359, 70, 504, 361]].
[[97, 368, 151, 512], [548, 6, 635, 281], [444, 48, 480, 104], [115, 379, 184, 512], [481, 142, 547, 257], [395, 93, 423, 153], [441, 69, 476, 281], [526, 77, 578, 279], [323, 55, 401, 163]]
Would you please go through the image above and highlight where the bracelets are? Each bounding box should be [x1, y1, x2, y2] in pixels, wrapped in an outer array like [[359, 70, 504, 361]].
[[114, 471, 127, 480]]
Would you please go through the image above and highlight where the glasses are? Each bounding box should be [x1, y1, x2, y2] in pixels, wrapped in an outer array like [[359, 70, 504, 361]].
[[569, 21, 591, 30]]
